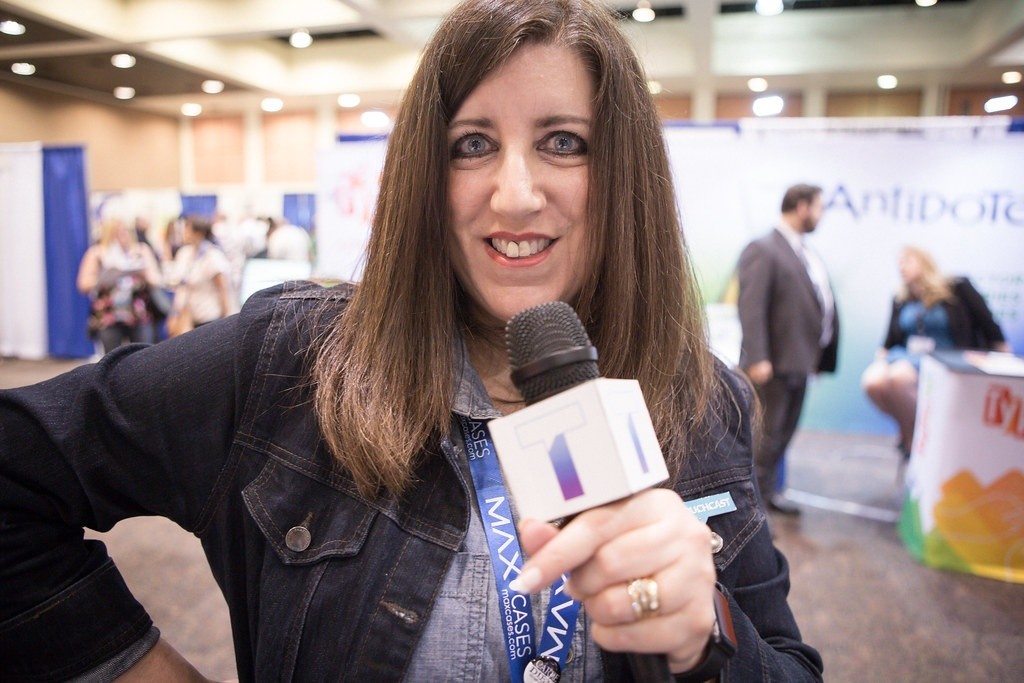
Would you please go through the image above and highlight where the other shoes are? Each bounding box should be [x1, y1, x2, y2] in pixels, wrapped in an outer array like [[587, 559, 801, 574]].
[[768, 494, 802, 514]]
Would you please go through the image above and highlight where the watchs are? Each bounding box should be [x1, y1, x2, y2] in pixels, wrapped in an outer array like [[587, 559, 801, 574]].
[[670, 585, 738, 683]]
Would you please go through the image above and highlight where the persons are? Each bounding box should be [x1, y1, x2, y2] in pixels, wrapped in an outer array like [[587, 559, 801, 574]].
[[0, 0, 825, 682], [862, 246, 1013, 462], [78, 192, 311, 368], [737, 184, 840, 515]]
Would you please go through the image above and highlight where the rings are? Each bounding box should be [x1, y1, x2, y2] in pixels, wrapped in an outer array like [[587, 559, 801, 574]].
[[628, 578, 659, 616]]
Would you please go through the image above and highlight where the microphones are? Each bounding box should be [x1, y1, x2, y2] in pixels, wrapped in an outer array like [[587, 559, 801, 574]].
[[486, 300, 672, 683]]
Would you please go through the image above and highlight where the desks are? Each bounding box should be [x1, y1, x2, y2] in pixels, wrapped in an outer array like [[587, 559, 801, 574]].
[[896, 350, 1024, 584]]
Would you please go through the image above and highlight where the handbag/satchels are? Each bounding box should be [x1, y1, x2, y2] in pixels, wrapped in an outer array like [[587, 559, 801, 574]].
[[165, 306, 194, 339]]
